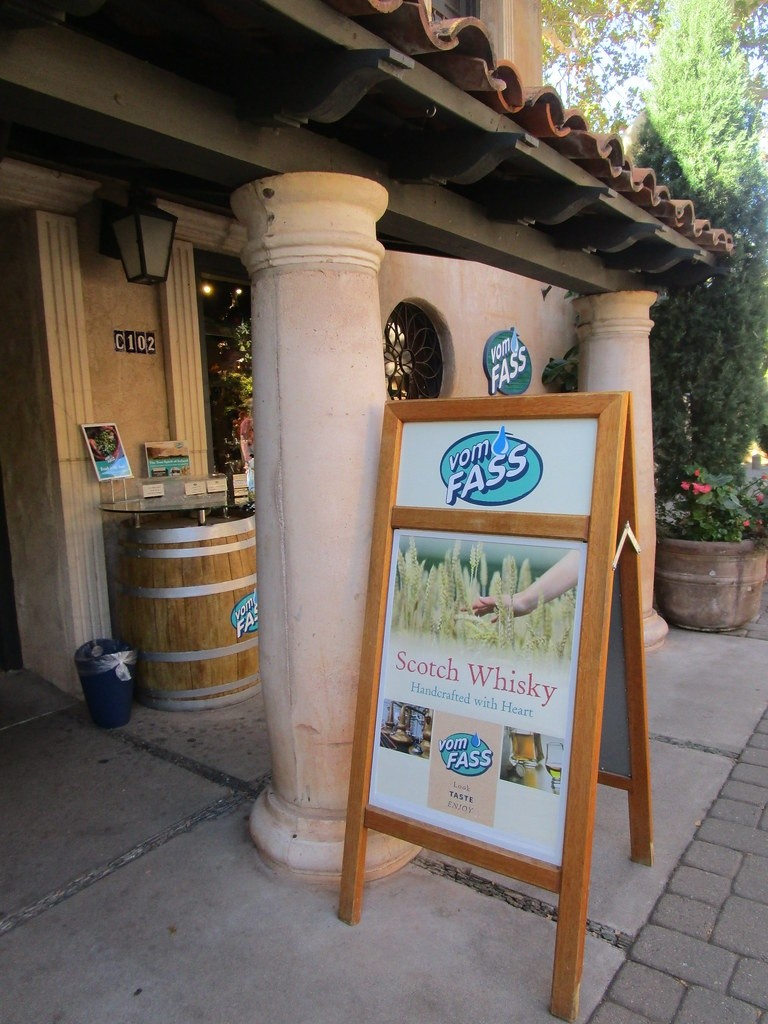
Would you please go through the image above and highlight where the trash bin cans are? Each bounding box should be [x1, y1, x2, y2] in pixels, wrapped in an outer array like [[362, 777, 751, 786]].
[[74, 637, 138, 731]]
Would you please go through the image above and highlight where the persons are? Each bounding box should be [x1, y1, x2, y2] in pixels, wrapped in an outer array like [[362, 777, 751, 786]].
[[460, 549, 579, 623]]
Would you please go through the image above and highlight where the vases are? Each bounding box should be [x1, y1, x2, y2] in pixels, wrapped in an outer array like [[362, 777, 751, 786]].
[[655, 537, 768, 632]]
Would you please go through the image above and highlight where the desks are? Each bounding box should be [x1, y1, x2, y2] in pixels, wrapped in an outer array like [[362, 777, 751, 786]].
[[98, 494, 254, 529]]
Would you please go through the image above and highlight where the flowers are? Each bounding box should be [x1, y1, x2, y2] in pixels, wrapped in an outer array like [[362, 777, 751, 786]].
[[654, 464, 768, 542]]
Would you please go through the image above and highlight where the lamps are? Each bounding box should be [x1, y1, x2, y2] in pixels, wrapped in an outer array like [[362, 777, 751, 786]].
[[98, 187, 178, 286]]
